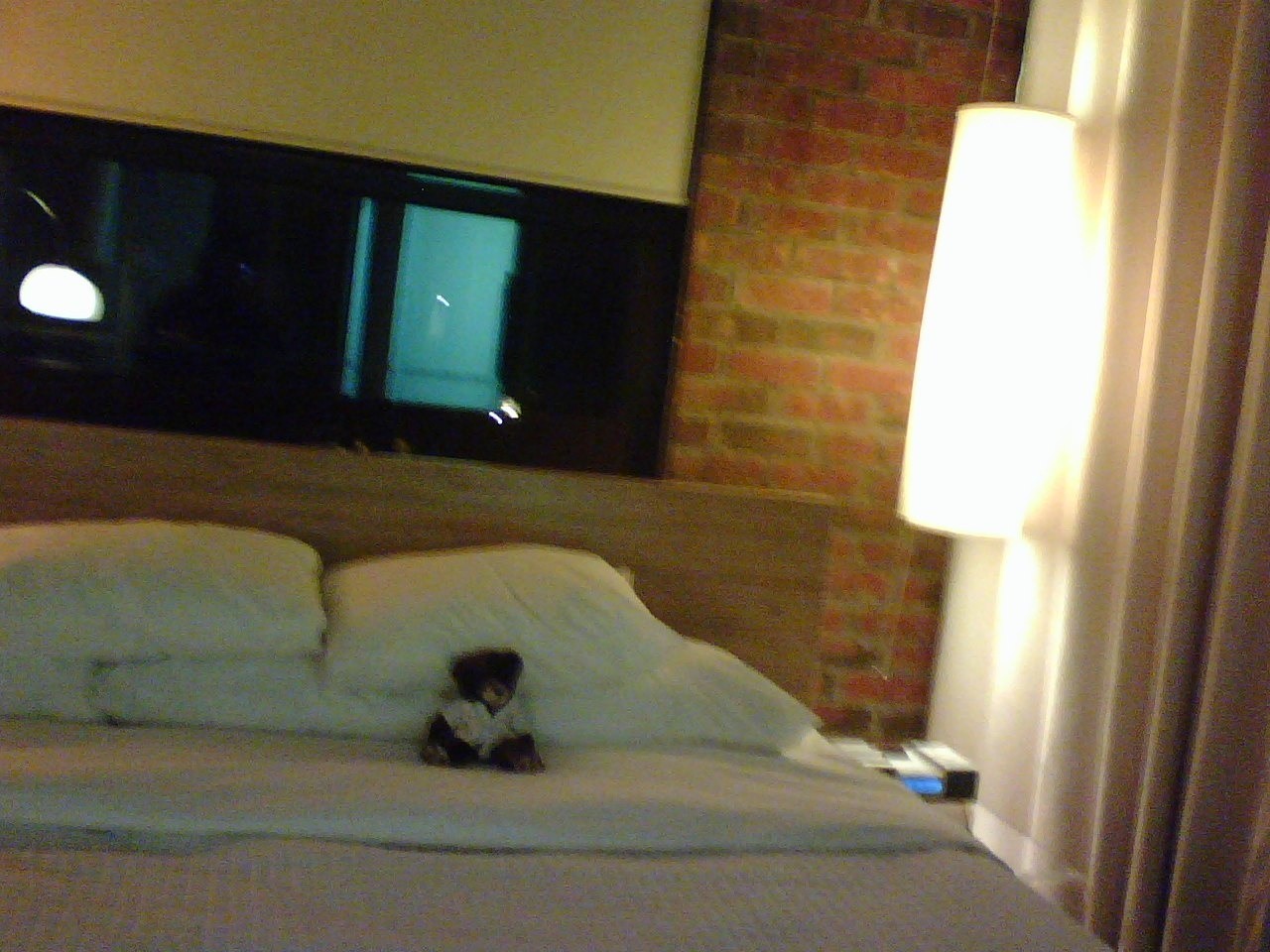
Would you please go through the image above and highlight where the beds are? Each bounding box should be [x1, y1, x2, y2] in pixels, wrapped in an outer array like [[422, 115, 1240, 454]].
[[1, 418, 1115, 950]]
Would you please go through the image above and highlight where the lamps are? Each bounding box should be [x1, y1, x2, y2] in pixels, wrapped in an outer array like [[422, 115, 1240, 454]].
[[0, 185, 105, 325], [895, 97, 1082, 537]]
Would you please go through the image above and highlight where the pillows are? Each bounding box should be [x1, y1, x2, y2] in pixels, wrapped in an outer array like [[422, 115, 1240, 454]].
[[1, 520, 822, 761]]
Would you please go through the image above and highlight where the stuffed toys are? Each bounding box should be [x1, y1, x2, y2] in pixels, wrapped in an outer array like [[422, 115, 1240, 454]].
[[419, 646, 546, 773]]
